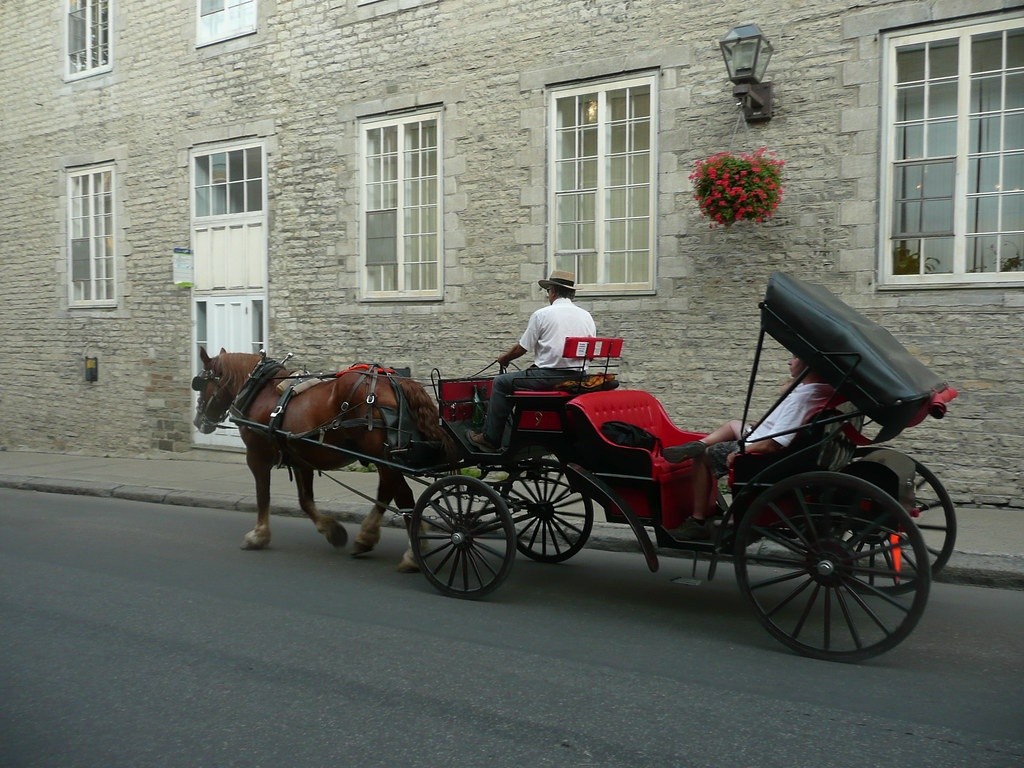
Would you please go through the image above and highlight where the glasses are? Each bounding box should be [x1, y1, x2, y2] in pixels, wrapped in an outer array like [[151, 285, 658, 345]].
[[547, 288, 550, 293]]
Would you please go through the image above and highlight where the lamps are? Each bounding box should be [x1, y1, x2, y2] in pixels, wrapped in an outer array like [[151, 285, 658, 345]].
[[719, 22, 774, 123]]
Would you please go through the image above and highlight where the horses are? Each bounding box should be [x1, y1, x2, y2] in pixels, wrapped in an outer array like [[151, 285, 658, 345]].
[[193, 346, 453, 572]]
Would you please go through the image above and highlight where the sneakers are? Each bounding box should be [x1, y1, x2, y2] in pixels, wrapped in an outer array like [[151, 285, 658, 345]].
[[663, 441, 706, 463], [465, 429, 500, 453], [668, 515, 710, 541]]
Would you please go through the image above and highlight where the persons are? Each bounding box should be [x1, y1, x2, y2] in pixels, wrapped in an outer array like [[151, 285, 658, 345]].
[[661, 352, 848, 541], [465, 271, 597, 453]]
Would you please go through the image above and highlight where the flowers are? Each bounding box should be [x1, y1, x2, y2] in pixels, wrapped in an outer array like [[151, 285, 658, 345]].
[[688, 145, 786, 229]]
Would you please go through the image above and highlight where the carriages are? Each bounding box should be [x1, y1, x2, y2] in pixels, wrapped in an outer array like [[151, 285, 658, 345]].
[[189, 273, 959, 664]]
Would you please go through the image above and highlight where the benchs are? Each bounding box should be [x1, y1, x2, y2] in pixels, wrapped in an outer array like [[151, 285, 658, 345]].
[[566, 390, 710, 481], [728, 395, 865, 487], [505, 336, 623, 404]]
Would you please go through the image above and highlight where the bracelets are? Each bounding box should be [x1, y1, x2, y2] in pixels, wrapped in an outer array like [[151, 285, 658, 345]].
[[734, 450, 740, 455]]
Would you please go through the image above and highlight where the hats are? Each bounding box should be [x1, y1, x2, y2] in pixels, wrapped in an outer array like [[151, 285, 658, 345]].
[[538, 270, 584, 290]]
